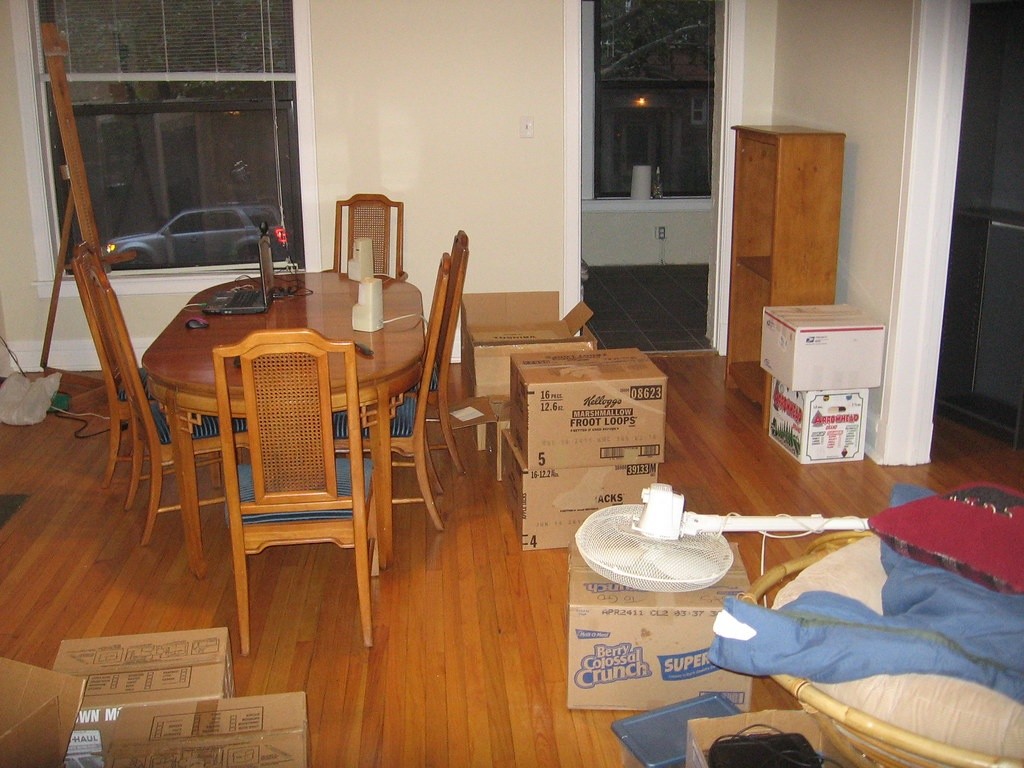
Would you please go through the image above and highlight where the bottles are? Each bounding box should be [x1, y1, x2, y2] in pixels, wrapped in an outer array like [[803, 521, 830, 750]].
[[653, 167, 663, 199]]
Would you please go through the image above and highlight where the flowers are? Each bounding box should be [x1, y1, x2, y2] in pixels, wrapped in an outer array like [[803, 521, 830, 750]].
[[403, 230, 469, 491]]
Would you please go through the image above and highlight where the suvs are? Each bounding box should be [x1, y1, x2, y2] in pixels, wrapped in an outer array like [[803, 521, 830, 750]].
[[106, 205, 288, 271]]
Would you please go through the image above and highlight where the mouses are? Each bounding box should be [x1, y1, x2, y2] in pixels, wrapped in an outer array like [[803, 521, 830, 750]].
[[185, 317, 209, 328]]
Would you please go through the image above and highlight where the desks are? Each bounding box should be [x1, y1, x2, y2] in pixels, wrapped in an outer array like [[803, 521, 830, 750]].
[[141, 273, 426, 578]]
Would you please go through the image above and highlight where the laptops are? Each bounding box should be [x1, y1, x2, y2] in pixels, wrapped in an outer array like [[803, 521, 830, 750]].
[[202, 231, 275, 315]]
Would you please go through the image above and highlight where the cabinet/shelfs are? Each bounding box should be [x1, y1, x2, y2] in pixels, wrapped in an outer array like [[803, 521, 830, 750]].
[[724, 124, 846, 428]]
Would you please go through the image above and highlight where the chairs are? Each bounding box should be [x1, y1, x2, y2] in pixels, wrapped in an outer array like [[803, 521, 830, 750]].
[[319, 194, 411, 282], [80, 241, 257, 558], [311, 252, 450, 531], [212, 328, 372, 655], [73, 241, 221, 488]]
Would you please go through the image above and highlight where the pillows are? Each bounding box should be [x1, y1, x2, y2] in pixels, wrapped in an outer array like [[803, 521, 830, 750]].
[[866, 481, 1023, 597]]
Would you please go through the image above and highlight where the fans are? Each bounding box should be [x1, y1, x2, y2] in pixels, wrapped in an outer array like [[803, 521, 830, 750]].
[[574, 483, 874, 591]]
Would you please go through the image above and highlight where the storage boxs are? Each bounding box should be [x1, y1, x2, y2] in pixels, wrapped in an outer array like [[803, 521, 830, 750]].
[[611, 692, 742, 768], [685, 710, 875, 768], [1, 627, 310, 768], [769, 376, 869, 463], [759, 304, 886, 391], [37, 365, 108, 412], [449, 291, 754, 710]]
